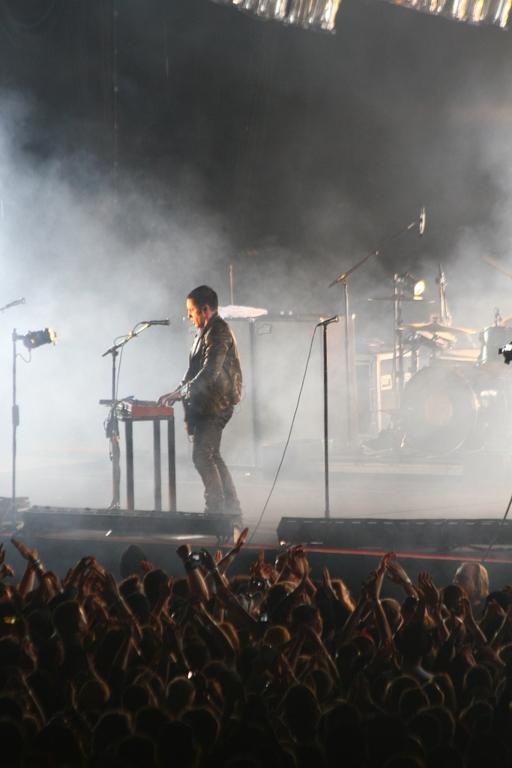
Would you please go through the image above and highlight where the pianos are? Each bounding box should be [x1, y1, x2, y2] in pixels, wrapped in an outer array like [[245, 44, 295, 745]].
[[113, 397, 174, 419]]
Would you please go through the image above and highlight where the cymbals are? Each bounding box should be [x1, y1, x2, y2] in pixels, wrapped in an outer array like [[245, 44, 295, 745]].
[[368, 294, 423, 301], [435, 330, 457, 341], [217, 304, 267, 322], [403, 321, 479, 335]]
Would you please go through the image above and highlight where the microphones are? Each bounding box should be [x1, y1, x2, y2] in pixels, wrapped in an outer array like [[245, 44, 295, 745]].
[[5, 297, 26, 309], [148, 319, 171, 326], [419, 207, 425, 235], [317, 315, 340, 327]]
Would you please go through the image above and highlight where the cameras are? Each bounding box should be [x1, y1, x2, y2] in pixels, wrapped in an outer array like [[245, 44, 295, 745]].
[[253, 579, 265, 592], [185, 550, 205, 570]]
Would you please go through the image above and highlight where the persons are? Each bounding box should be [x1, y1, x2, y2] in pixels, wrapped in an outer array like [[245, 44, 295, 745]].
[[156, 285, 242, 515], [2, 528, 512, 766]]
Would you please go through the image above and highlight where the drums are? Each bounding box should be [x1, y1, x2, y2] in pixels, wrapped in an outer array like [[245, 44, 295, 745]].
[[479, 325, 512, 376], [392, 364, 508, 463], [413, 332, 448, 350]]
